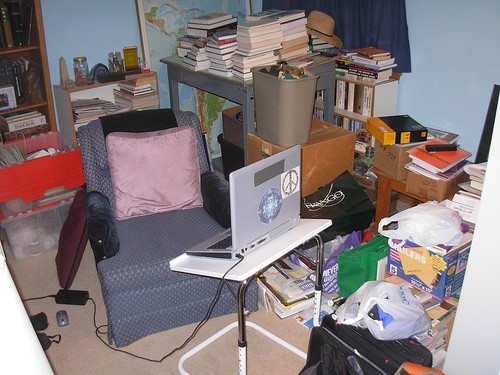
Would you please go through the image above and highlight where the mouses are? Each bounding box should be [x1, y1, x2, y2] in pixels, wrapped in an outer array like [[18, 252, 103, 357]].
[[56, 310, 68, 326]]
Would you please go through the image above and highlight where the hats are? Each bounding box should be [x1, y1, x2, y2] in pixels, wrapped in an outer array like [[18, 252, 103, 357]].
[[306, 11, 342, 48]]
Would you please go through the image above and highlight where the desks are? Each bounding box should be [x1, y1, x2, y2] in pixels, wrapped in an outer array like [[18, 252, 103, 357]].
[[370, 166, 430, 235], [169, 219, 334, 375], [159, 55, 336, 166]]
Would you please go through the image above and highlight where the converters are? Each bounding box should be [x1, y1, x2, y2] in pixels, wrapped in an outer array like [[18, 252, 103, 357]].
[[55, 289, 89, 305]]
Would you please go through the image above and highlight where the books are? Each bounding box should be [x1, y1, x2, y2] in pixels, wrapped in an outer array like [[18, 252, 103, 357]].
[[0, 0, 50, 168], [71, 75, 159, 123], [176, 9, 488, 368]]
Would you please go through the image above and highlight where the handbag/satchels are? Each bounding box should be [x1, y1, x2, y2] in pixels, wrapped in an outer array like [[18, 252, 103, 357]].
[[300, 312, 433, 375], [338, 236, 389, 297], [336, 280, 432, 340], [379, 201, 463, 250], [300, 169, 376, 249], [322, 230, 362, 295]]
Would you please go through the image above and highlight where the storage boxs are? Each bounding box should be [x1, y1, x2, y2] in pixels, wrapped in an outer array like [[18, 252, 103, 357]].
[[386, 227, 476, 302], [0, 85, 17, 111], [217, 65, 470, 202], [0, 132, 87, 261]]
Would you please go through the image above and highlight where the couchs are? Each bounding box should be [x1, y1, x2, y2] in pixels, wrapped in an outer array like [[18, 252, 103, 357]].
[[78, 110, 259, 348]]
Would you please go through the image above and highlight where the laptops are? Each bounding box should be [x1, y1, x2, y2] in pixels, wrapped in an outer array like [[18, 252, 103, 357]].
[[186, 144, 301, 258]]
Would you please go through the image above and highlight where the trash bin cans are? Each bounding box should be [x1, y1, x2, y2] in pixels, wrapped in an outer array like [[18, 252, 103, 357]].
[[250, 65, 320, 148]]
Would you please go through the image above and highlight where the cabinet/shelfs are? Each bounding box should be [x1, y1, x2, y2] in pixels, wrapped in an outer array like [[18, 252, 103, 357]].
[[314, 74, 401, 155], [53, 70, 159, 145], [0, 0, 57, 132]]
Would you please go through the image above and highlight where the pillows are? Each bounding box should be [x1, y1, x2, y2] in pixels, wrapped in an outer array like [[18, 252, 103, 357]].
[[106, 125, 203, 221], [55, 189, 89, 291]]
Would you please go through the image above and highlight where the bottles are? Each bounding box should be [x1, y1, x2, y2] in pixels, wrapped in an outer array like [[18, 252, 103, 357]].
[[107, 52, 123, 75], [73, 56, 90, 86]]
